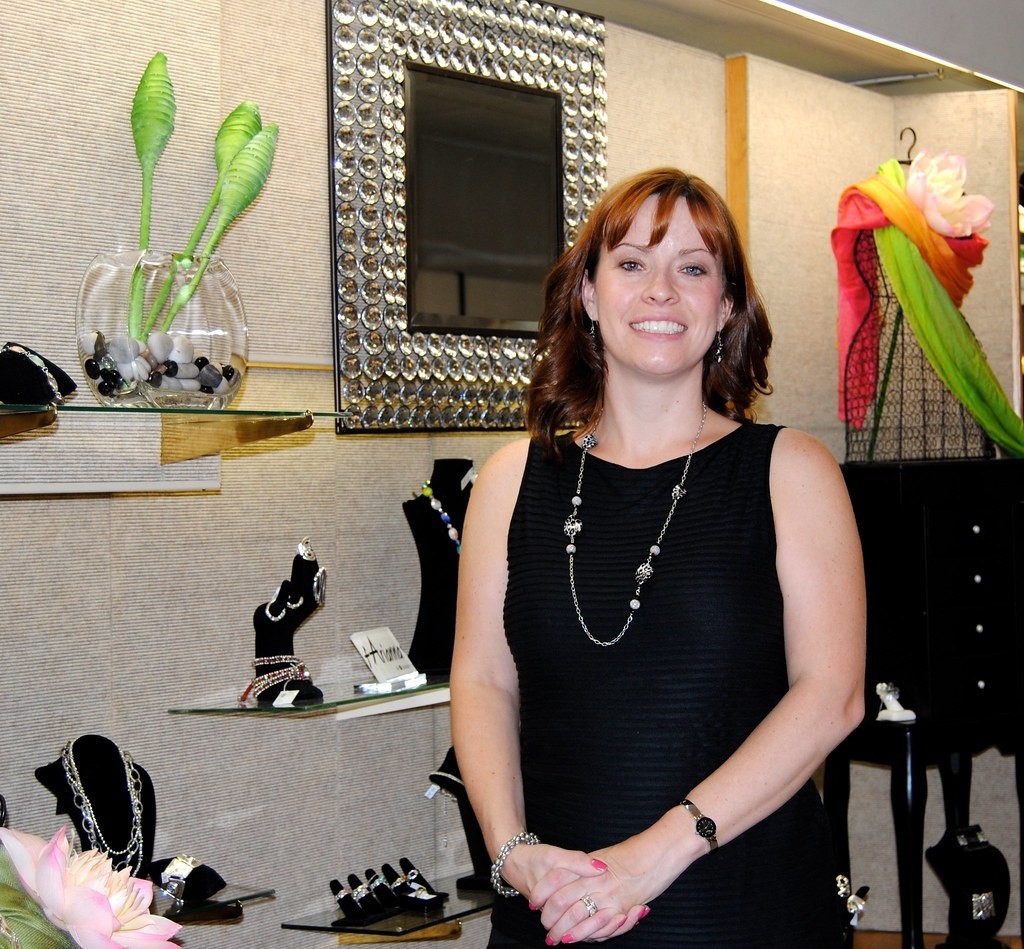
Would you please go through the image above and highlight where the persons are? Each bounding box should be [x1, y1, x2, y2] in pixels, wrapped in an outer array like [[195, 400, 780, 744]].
[[447, 168, 867, 949]]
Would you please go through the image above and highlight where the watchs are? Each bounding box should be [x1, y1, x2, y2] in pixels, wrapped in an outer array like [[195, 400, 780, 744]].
[[682, 797, 719, 852]]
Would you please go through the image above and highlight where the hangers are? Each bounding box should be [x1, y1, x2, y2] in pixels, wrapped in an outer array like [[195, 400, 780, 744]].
[[895, 127, 918, 165]]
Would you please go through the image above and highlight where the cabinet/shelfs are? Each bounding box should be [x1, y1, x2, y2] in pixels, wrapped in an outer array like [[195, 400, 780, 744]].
[[0, 400, 497, 939]]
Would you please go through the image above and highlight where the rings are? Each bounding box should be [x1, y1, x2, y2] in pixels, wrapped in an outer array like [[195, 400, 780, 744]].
[[265, 600, 286, 623], [286, 596, 304, 609], [314, 567, 327, 607], [579, 896, 598, 917], [336, 868, 426, 903]]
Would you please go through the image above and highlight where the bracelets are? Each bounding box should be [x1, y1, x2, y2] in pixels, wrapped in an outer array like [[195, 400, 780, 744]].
[[0, 346, 64, 403], [491, 832, 541, 897], [249, 656, 313, 701]]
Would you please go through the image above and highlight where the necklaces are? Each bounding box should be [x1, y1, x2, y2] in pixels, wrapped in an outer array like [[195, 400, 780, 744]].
[[564, 400, 707, 645], [422, 479, 461, 553], [61, 736, 144, 878]]
